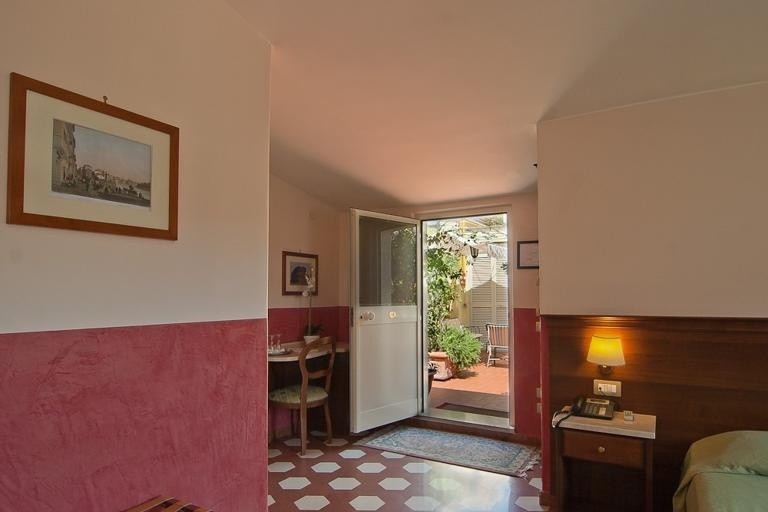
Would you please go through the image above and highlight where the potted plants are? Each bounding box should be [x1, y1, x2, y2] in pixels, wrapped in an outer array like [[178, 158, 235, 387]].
[[303, 323, 325, 345], [429, 326, 481, 381], [427, 354, 437, 394]]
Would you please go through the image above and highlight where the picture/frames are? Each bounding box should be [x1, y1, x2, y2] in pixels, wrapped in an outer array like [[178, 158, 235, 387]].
[[6, 72, 181, 241], [517, 240, 539, 269], [282, 250, 319, 296]]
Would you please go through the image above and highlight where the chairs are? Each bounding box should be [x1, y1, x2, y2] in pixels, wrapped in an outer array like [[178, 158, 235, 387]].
[[486, 324, 509, 368], [268, 336, 336, 457]]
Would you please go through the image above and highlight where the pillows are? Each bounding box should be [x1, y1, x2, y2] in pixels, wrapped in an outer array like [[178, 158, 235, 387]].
[[693, 429, 768, 469]]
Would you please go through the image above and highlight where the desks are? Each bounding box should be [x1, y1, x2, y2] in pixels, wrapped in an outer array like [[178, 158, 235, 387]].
[[268, 340, 349, 362], [550, 405, 657, 510]]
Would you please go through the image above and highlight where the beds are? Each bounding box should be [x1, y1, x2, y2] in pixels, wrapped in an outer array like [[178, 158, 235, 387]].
[[689, 443, 768, 512]]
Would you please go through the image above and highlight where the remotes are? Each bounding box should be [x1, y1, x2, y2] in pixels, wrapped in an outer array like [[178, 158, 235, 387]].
[[624, 411, 633, 421]]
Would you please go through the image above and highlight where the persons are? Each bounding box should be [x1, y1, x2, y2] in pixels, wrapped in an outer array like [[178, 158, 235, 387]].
[[123, 185, 139, 198]]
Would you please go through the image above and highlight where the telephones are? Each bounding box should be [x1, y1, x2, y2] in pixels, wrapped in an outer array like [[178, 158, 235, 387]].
[[572, 396, 616, 420]]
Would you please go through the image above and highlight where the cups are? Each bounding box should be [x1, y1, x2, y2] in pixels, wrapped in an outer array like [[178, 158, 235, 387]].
[[268, 332, 284, 350]]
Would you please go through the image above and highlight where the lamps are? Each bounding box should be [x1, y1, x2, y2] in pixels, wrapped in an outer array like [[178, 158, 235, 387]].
[[586, 333, 625, 375]]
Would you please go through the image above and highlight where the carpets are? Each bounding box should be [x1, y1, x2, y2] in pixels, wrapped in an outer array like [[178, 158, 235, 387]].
[[353, 423, 541, 480]]
